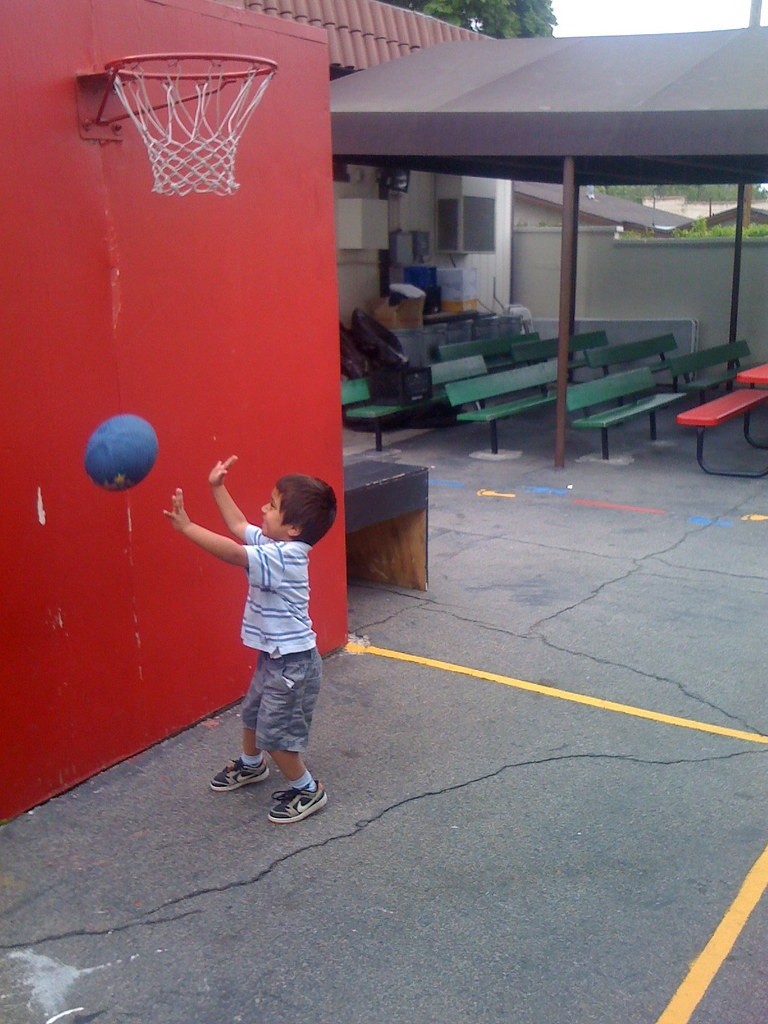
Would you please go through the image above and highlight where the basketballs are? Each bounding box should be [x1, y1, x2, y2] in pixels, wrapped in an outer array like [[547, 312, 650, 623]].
[[84, 414, 158, 491]]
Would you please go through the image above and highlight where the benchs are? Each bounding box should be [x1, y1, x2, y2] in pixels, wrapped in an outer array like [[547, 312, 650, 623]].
[[339, 331, 768, 477]]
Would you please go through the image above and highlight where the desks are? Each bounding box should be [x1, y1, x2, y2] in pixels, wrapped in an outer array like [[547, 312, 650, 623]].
[[340, 460, 430, 596]]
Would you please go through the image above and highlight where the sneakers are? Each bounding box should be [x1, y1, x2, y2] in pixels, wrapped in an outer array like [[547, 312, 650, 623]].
[[210, 757, 269, 791], [267, 780, 328, 824]]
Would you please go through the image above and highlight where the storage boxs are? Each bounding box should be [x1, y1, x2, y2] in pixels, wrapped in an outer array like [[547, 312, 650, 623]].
[[437, 267, 479, 312]]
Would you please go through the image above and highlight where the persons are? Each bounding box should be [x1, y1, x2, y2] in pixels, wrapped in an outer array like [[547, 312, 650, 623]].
[[162, 455, 338, 824]]
